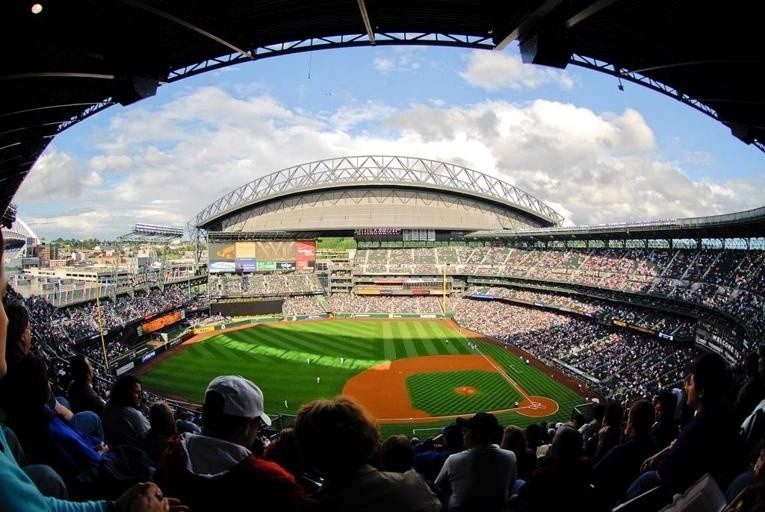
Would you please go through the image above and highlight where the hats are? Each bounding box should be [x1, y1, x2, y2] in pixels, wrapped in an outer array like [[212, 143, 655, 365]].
[[456, 413, 501, 439], [203, 375, 272, 427]]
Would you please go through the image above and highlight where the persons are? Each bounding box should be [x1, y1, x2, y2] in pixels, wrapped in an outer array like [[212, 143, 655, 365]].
[[0, 228, 765, 512]]
